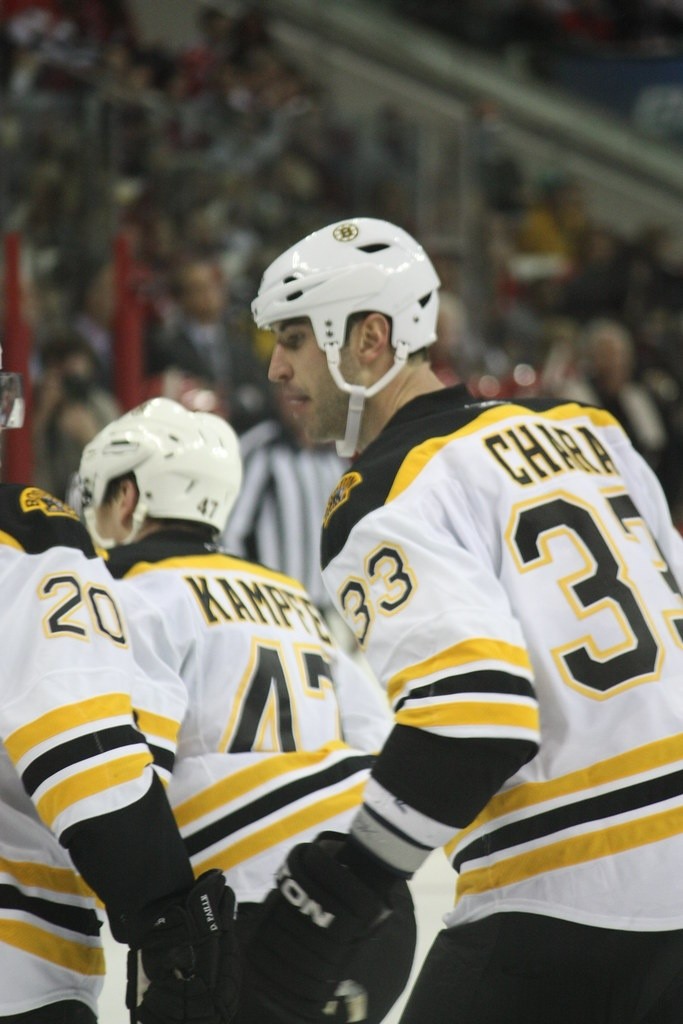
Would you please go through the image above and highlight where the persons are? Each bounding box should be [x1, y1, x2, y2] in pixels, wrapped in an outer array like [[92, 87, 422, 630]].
[[249, 216, 683, 1024], [74, 397, 417, 1024], [0, 341, 247, 1024], [0, 0, 682, 537]]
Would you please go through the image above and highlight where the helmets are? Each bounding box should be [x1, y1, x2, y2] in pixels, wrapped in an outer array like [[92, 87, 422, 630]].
[[72, 397, 242, 535], [250, 216, 443, 357]]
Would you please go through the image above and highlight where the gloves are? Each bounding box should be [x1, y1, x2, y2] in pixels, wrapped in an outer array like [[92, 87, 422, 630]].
[[122, 871, 249, 1024], [246, 833, 417, 1024]]
